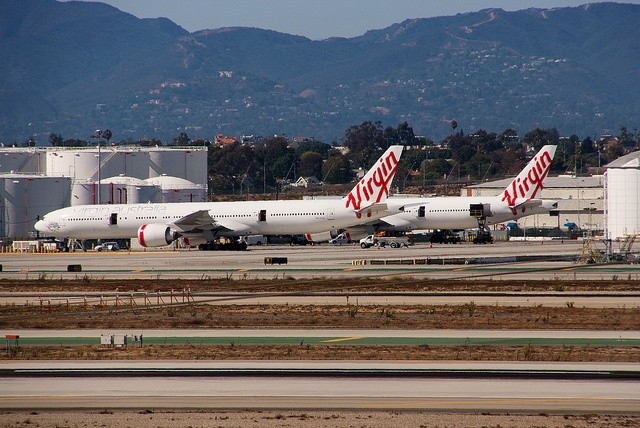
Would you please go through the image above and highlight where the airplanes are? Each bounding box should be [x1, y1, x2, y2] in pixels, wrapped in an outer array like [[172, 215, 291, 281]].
[[304, 143, 561, 242], [33, 143, 407, 250]]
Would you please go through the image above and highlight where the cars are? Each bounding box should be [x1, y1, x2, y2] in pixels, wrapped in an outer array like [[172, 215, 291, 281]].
[[331, 231, 351, 243]]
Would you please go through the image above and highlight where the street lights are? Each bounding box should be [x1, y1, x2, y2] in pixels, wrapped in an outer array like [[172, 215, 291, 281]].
[[96, 129, 102, 204]]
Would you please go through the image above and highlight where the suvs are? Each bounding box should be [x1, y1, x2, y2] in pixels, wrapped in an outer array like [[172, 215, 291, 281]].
[[94, 241, 120, 251]]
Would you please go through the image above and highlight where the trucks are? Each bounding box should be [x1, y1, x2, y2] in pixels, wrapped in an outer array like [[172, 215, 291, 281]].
[[288, 233, 315, 245], [359, 234, 411, 248], [236, 234, 264, 245]]
